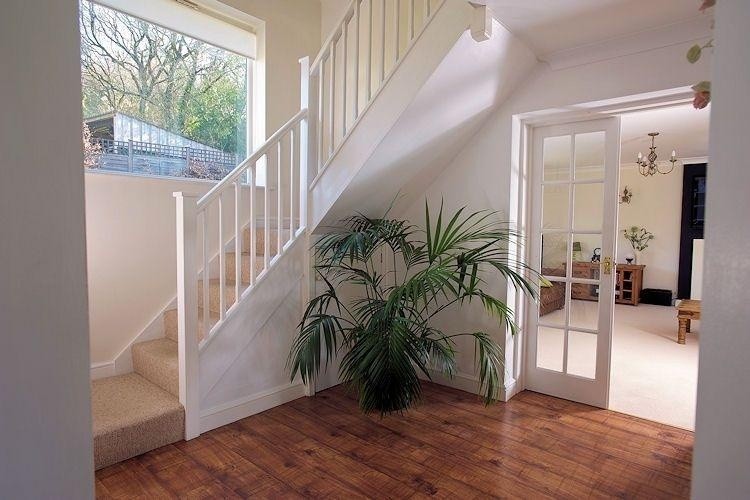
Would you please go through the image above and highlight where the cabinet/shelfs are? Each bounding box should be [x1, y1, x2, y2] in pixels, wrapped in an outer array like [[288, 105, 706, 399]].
[[562, 260, 645, 307]]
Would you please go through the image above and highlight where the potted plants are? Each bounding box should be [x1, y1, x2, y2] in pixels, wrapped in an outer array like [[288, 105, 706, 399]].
[[280, 184, 555, 424]]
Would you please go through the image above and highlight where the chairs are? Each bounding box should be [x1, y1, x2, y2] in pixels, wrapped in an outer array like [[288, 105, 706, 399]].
[[540, 266, 568, 314]]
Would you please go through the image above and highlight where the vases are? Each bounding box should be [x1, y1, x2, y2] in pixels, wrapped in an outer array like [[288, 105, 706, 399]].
[[633, 249, 640, 264]]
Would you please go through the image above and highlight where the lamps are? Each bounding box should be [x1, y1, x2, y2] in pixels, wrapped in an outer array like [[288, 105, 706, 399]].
[[619, 185, 634, 207], [634, 130, 678, 179]]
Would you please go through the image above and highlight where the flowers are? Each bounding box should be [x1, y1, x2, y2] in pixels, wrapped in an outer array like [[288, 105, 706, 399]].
[[620, 226, 655, 250]]
